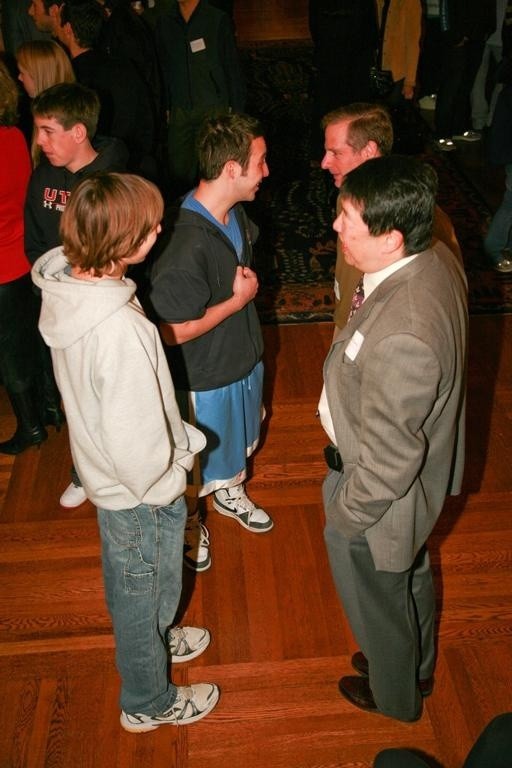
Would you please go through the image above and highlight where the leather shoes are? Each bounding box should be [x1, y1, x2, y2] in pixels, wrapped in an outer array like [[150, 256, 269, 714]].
[[338, 675, 380, 713], [352, 651, 432, 696]]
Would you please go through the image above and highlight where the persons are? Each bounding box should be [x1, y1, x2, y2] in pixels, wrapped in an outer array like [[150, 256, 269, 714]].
[[317, 156, 468, 723], [30, 172, 221, 736], [1, 1, 512, 573]]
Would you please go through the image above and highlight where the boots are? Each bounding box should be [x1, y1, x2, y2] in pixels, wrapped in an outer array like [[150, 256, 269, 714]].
[[1, 366, 65, 455]]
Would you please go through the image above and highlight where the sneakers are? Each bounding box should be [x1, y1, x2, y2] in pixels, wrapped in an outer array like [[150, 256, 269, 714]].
[[211, 482, 274, 533], [165, 624, 210, 664], [120, 681, 220, 733], [182, 506, 212, 572], [59, 482, 88, 510]]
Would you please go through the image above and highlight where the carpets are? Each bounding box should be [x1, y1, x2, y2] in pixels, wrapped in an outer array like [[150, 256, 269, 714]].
[[224, 34, 512, 324]]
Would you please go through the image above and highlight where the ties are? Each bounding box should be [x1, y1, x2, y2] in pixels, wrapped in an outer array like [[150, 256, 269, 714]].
[[346, 273, 366, 325]]
